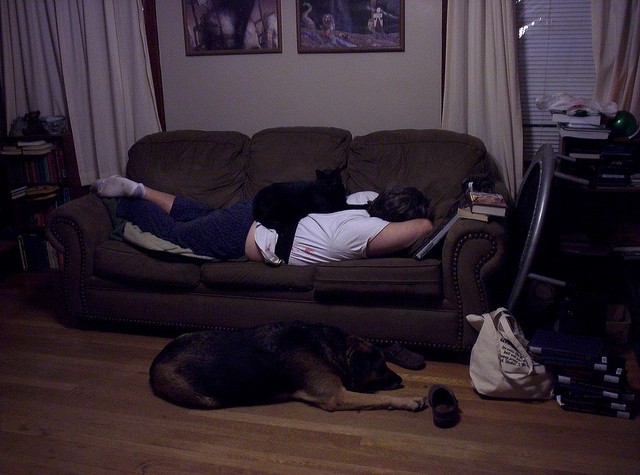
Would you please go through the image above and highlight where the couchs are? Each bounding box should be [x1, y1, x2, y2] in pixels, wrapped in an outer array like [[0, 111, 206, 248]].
[[43, 124, 515, 353]]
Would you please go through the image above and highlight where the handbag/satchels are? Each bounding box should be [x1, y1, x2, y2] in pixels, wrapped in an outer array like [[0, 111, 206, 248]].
[[466, 307, 550, 400]]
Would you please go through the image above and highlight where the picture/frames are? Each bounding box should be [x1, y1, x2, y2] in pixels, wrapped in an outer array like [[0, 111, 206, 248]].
[[182, 0, 283, 57], [296, 0, 406, 54]]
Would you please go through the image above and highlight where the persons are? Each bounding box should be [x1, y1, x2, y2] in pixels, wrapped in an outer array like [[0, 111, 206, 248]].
[[92, 175, 433, 267]]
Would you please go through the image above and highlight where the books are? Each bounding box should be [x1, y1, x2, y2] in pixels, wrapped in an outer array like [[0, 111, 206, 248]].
[[531, 330, 638, 419], [10, 184, 28, 200], [558, 234, 589, 254], [469, 203, 508, 218], [608, 228, 640, 261], [26, 186, 61, 200], [457, 197, 489, 222], [569, 151, 599, 160], [0, 145, 21, 154], [555, 112, 612, 141], [630, 174, 640, 188], [22, 143, 54, 155], [413, 201, 463, 259]]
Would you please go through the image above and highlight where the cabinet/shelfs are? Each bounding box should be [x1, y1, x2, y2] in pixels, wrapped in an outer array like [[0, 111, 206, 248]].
[[558, 124, 639, 163], [1, 132, 84, 269]]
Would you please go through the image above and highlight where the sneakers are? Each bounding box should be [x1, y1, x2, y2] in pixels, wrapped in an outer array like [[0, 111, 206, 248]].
[[428, 384, 458, 428], [383, 342, 424, 369]]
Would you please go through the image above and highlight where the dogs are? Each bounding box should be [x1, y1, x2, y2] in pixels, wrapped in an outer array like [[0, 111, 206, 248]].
[[149, 319, 428, 412]]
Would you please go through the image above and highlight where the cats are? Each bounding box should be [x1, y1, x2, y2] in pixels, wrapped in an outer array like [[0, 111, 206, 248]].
[[252, 166, 348, 229]]
